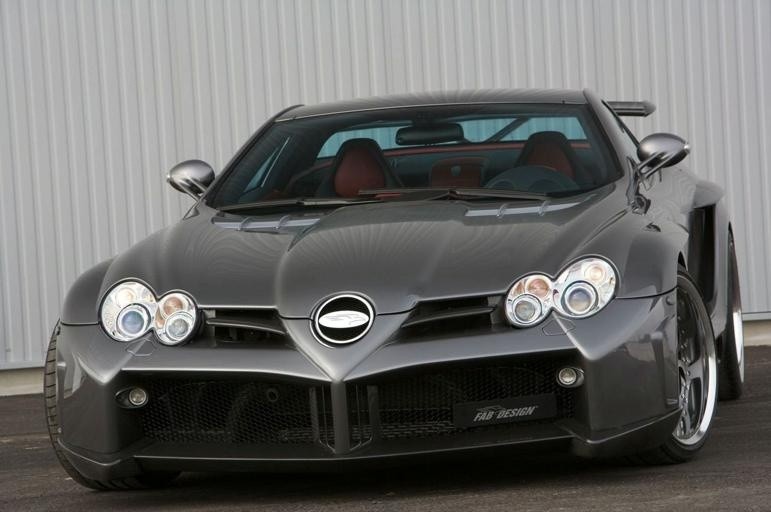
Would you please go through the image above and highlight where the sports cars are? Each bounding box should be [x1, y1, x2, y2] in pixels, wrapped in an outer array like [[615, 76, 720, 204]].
[[43, 85, 745, 493]]
